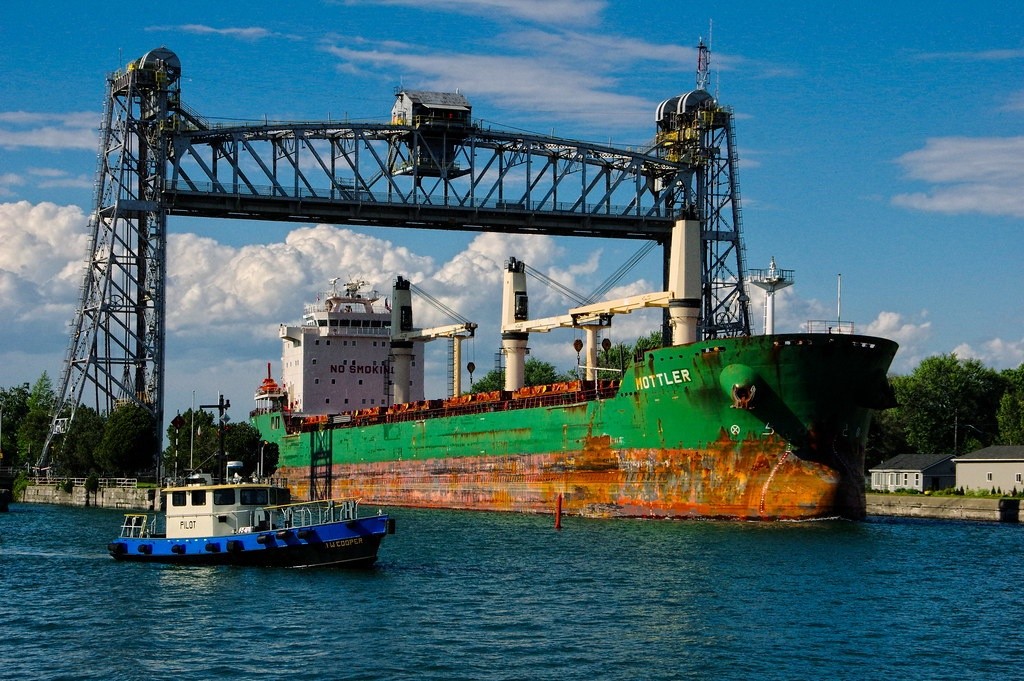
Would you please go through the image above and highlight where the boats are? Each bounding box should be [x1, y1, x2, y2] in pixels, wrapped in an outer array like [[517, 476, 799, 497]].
[[252, 197, 900, 522], [108, 390, 394, 563]]
